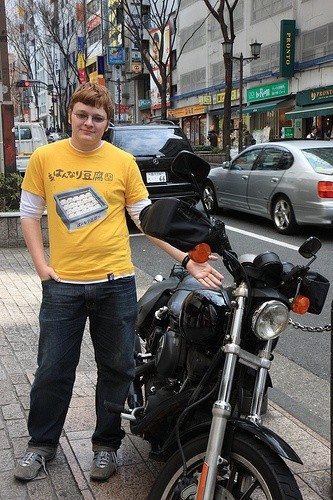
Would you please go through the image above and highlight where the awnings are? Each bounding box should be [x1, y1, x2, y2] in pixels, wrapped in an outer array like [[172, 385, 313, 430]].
[[285, 102, 333, 120], [236, 98, 292, 115]]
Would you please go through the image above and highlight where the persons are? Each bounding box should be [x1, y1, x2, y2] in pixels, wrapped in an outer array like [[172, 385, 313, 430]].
[[210, 133, 218, 147], [307, 127, 318, 138], [46, 124, 60, 134], [13, 82, 225, 481], [152, 33, 159, 60], [244, 131, 253, 148]]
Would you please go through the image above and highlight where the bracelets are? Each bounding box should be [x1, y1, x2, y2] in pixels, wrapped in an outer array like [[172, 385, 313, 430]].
[[181, 255, 190, 268]]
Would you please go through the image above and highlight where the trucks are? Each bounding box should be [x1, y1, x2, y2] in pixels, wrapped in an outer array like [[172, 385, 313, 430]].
[[14, 120, 50, 173]]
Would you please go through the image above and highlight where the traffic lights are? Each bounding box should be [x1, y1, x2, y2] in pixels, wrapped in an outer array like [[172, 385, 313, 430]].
[[16, 81, 30, 87]]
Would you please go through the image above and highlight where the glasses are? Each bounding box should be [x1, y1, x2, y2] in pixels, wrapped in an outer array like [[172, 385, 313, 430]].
[[74, 111, 110, 123]]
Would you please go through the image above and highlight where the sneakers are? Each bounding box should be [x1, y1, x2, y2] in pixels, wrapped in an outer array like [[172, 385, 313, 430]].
[[89, 450, 118, 480], [14, 450, 50, 481]]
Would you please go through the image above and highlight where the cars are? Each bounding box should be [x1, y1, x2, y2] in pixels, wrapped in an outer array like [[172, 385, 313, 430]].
[[48, 132, 70, 144], [201, 139, 333, 236]]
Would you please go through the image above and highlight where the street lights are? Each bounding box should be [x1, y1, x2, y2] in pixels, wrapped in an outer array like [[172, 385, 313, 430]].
[[221, 38, 263, 154]]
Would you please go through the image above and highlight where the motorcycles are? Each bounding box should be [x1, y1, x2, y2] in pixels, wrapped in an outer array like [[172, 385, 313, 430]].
[[124, 149, 330, 500]]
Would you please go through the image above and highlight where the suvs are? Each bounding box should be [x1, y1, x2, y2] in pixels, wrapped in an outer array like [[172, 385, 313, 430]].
[[103, 118, 201, 224]]
